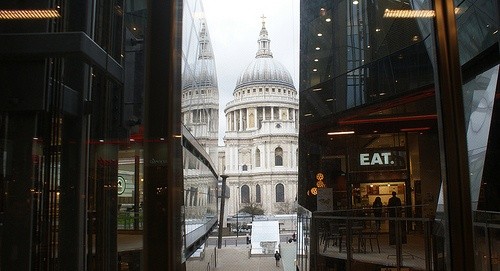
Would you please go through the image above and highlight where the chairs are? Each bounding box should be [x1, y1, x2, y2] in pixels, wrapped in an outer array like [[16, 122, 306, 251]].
[[322, 220, 381, 254]]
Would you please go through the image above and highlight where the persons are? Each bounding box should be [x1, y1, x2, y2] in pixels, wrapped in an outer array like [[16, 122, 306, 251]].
[[373, 197, 382, 231], [292, 232, 295, 239], [389, 191, 401, 206], [275, 251, 281, 267]]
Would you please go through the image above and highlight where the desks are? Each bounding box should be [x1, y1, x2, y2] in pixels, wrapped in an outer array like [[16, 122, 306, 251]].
[[339, 226, 362, 253]]
